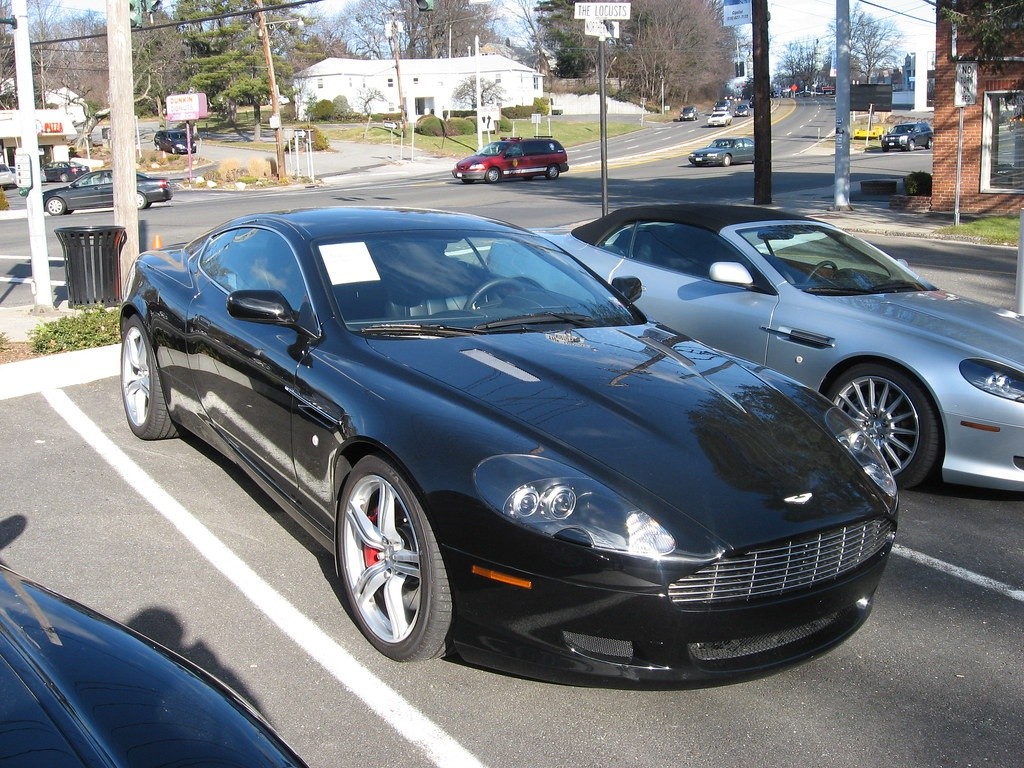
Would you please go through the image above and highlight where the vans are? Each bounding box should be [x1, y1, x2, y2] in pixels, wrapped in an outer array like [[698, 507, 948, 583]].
[[154, 130, 197, 154]]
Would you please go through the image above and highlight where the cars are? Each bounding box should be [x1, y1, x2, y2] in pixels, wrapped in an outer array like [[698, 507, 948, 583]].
[[800, 91, 811, 97], [0, 564, 311, 768], [688, 137, 754, 167], [0, 163, 15, 185], [119, 206, 900, 690], [708, 111, 733, 128], [734, 104, 751, 117], [8, 167, 47, 184], [749, 95, 755, 108], [41, 161, 90, 182], [771, 89, 782, 98], [452, 136, 569, 184], [270, 95, 290, 105], [42, 169, 174, 216], [484, 204, 1024, 492], [712, 100, 733, 111]]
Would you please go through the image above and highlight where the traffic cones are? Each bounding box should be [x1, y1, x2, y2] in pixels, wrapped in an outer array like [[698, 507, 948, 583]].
[[151, 234, 164, 250]]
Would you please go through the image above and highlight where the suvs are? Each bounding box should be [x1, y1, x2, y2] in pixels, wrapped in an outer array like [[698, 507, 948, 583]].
[[881, 121, 933, 152], [679, 106, 698, 121]]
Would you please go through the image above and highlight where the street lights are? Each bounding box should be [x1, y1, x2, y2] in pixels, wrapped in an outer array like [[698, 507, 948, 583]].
[[260, 17, 305, 180]]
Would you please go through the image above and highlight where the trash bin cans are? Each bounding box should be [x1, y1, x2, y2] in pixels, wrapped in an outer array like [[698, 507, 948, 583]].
[[53, 226, 126, 311]]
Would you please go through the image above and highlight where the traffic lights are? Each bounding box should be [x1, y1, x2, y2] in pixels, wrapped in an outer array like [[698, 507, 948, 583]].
[[129, 0, 143, 28], [145, 0, 160, 13], [416, 0, 434, 12]]
